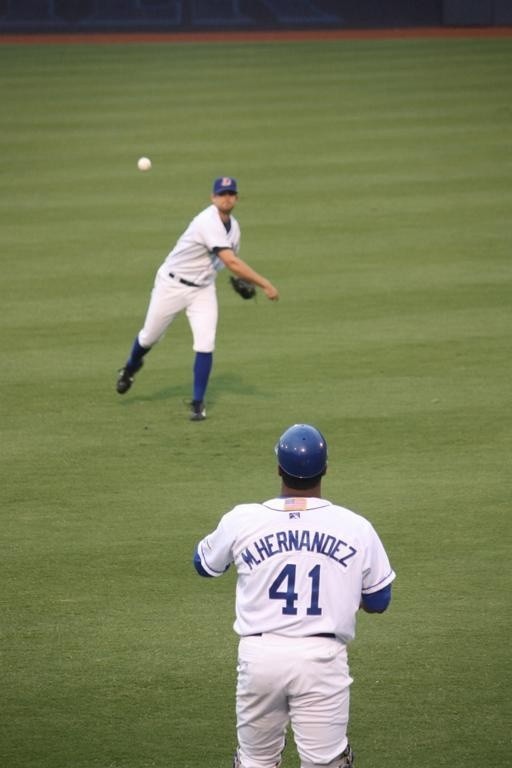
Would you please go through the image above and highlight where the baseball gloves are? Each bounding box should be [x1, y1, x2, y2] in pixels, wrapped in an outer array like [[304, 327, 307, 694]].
[[230, 278, 256, 298]]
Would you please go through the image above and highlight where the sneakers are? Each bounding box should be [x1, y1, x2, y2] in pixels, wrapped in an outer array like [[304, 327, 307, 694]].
[[189, 398, 208, 421], [116, 360, 145, 394]]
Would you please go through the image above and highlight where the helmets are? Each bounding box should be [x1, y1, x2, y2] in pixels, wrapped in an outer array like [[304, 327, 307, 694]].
[[273, 423, 329, 480]]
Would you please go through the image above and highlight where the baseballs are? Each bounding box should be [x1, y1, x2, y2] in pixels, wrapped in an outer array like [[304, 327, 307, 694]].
[[138, 155, 152, 172]]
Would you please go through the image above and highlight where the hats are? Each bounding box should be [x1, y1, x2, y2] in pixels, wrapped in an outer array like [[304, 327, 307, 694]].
[[212, 175, 239, 195]]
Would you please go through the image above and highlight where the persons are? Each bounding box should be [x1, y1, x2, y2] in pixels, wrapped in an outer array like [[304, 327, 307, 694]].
[[192, 420, 397, 768], [113, 175, 282, 422]]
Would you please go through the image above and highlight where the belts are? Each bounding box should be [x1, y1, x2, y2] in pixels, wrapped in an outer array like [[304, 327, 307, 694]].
[[249, 631, 336, 639], [169, 272, 204, 288]]
